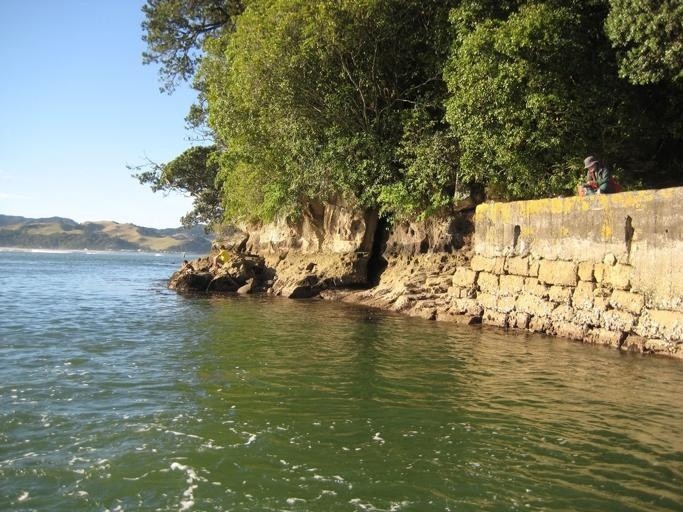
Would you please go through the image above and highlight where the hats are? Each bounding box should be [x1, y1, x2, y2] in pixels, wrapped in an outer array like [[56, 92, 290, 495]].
[[584, 156, 599, 168]]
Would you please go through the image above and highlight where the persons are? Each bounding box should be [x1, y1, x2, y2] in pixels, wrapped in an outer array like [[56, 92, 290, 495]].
[[180, 259, 194, 274], [184, 264, 192, 274], [577, 155, 621, 196]]
